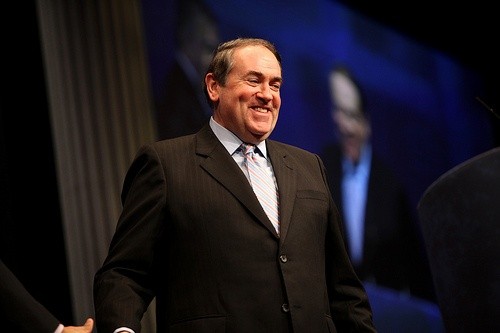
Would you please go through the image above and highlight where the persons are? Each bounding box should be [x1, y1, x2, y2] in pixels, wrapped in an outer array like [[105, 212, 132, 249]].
[[152, 0, 221, 143], [0, 261, 95, 333], [324, 65, 437, 305], [93, 38, 377, 333]]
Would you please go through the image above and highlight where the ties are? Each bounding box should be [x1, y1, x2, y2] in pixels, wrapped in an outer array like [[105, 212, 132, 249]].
[[241, 143, 280, 238]]
